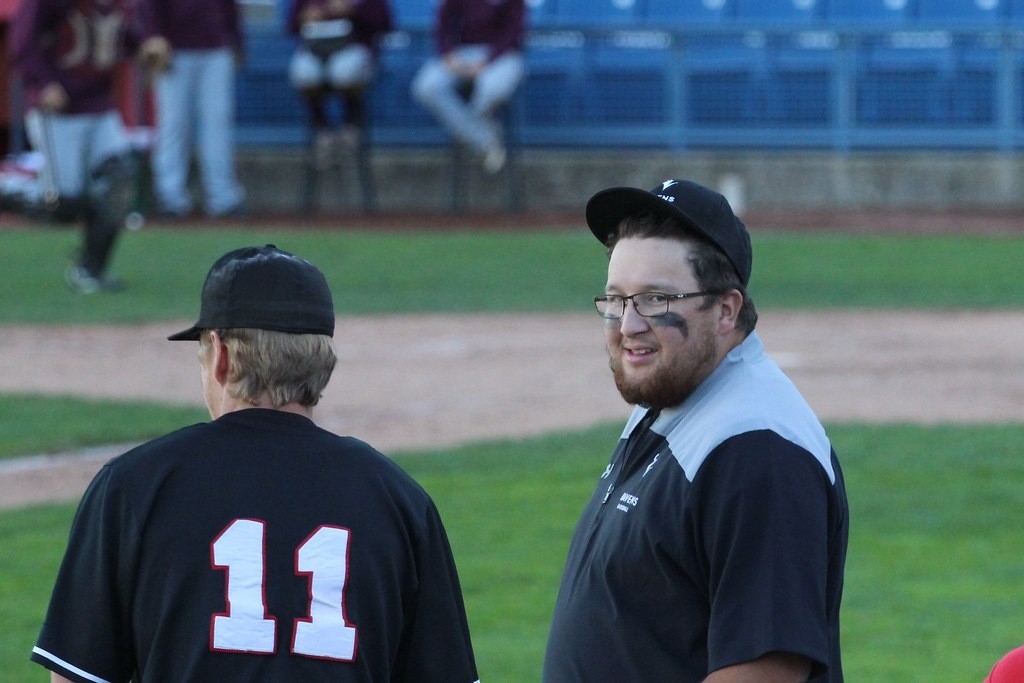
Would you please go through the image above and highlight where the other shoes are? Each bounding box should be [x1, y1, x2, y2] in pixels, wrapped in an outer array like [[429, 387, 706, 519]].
[[311, 124, 361, 170], [69, 264, 107, 299], [483, 123, 506, 173]]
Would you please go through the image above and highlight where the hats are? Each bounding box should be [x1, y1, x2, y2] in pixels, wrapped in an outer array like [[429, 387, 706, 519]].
[[585, 178, 753, 288], [168, 244, 334, 341]]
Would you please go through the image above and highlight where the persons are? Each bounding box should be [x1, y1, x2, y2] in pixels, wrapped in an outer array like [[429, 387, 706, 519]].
[[544, 179, 851, 683], [0, 1, 528, 293], [29, 242, 480, 683]]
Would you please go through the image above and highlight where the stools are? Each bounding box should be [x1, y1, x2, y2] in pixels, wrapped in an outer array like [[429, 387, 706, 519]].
[[451, 83, 523, 215], [304, 81, 378, 217]]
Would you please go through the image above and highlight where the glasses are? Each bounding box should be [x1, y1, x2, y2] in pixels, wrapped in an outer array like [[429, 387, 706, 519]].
[[594, 290, 719, 319]]
